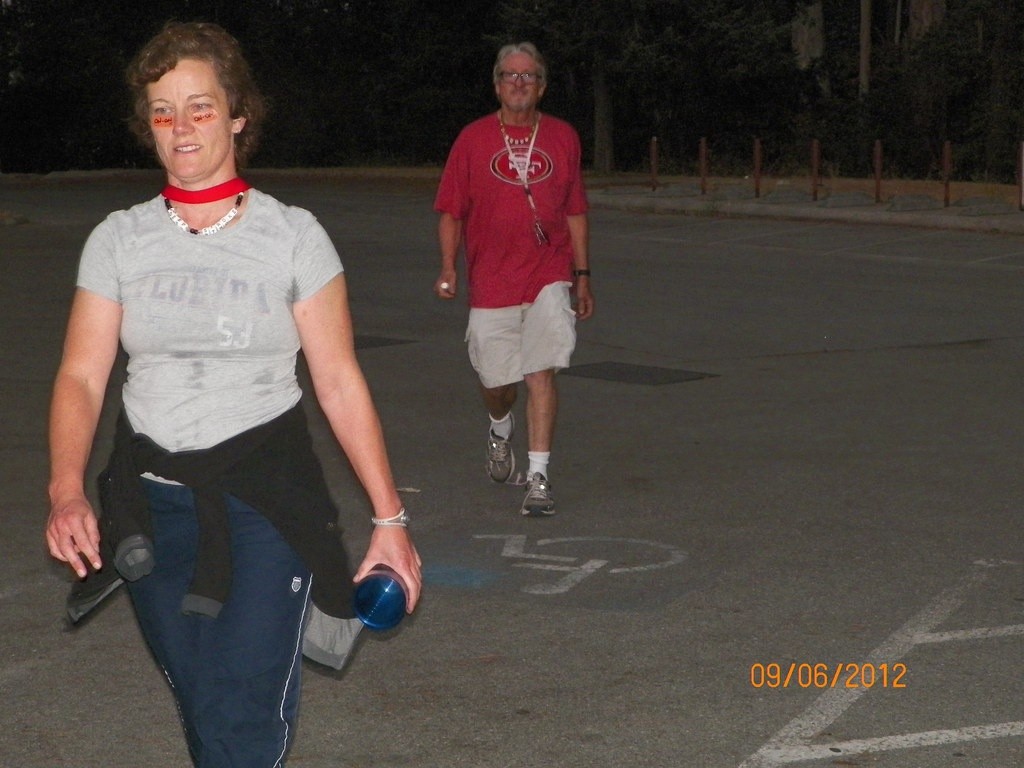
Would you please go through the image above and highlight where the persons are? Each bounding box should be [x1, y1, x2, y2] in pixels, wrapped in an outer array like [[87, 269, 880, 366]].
[[43, 16, 426, 768], [431, 37, 599, 518]]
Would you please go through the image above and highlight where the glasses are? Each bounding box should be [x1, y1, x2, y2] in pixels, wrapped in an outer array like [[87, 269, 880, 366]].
[[498, 71, 542, 84]]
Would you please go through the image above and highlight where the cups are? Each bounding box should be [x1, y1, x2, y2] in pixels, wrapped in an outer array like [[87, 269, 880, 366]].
[[355, 563, 410, 630]]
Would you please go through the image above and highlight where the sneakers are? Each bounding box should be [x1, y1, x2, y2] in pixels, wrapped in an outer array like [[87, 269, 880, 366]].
[[487, 411, 516, 483], [520, 472, 556, 515]]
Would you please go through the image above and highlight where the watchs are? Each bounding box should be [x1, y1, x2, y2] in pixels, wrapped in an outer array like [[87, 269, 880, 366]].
[[364, 507, 413, 529]]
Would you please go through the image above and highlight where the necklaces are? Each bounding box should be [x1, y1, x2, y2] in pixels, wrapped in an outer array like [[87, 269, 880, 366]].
[[161, 191, 243, 238], [499, 113, 537, 145]]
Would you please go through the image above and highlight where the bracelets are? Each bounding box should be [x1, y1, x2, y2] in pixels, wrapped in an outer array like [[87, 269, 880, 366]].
[[573, 268, 593, 277]]
